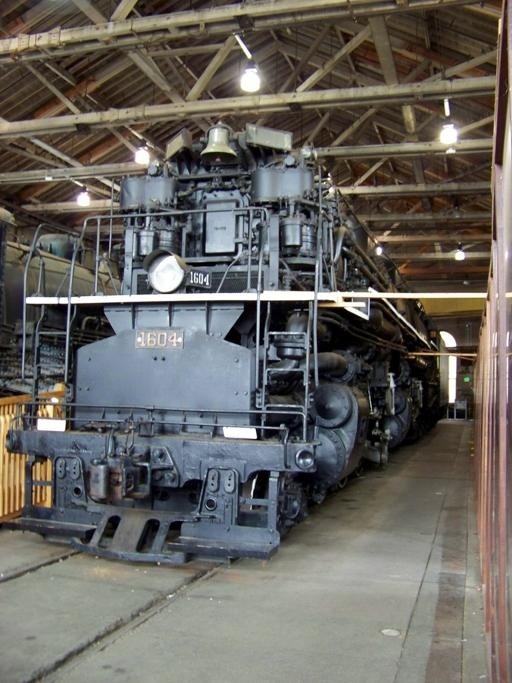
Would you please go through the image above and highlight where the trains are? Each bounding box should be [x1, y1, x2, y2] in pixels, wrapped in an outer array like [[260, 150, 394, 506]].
[[3, 123, 442, 570]]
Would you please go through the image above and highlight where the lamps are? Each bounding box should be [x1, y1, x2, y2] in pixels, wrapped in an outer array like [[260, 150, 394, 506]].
[[440, 117, 458, 145], [76, 185, 90, 207], [240, 59, 261, 92], [454, 241, 465, 261], [134, 138, 149, 164]]
